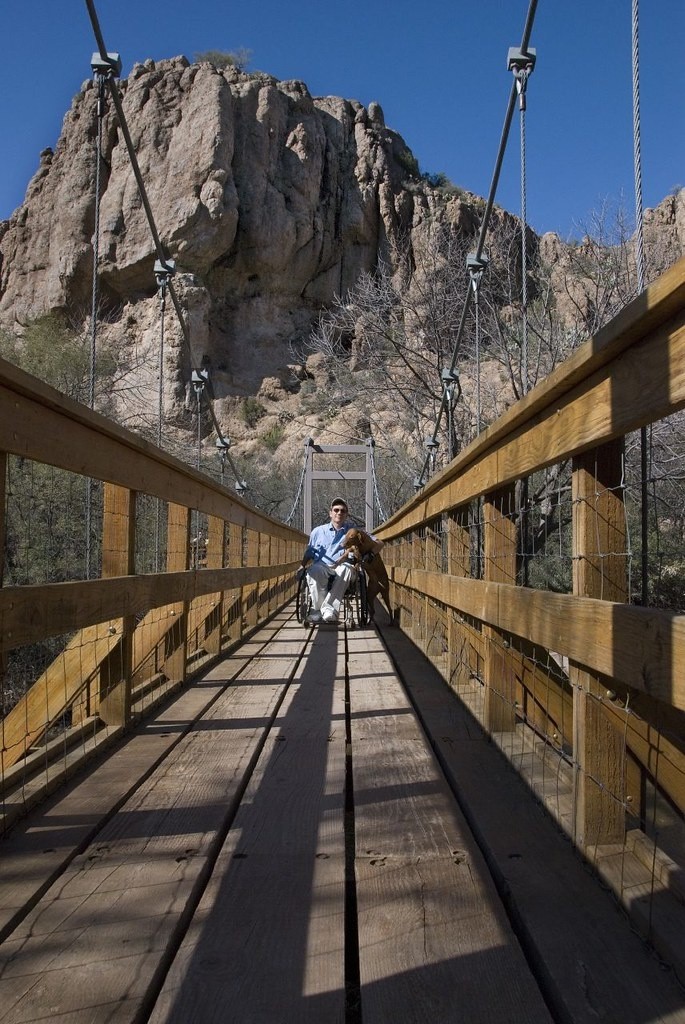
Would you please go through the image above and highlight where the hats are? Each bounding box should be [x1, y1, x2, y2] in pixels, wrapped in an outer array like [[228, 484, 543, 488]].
[[331, 497, 348, 510]]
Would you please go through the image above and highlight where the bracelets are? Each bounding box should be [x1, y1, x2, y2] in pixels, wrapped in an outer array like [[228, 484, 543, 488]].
[[369, 551, 376, 558]]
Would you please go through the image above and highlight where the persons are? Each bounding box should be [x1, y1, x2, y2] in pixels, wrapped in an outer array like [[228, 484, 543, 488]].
[[298, 497, 384, 622], [191, 531, 208, 566]]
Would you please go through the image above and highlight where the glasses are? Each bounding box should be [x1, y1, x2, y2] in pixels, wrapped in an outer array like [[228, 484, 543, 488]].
[[332, 509, 348, 513]]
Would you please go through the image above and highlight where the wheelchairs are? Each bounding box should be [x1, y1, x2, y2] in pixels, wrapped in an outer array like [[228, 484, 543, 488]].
[[296, 550, 367, 629]]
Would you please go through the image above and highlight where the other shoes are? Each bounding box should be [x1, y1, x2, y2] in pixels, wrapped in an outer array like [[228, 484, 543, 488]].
[[324, 613, 336, 622], [308, 612, 322, 622]]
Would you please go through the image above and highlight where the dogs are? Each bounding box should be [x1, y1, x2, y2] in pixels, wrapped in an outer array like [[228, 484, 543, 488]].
[[335, 527, 394, 627]]
